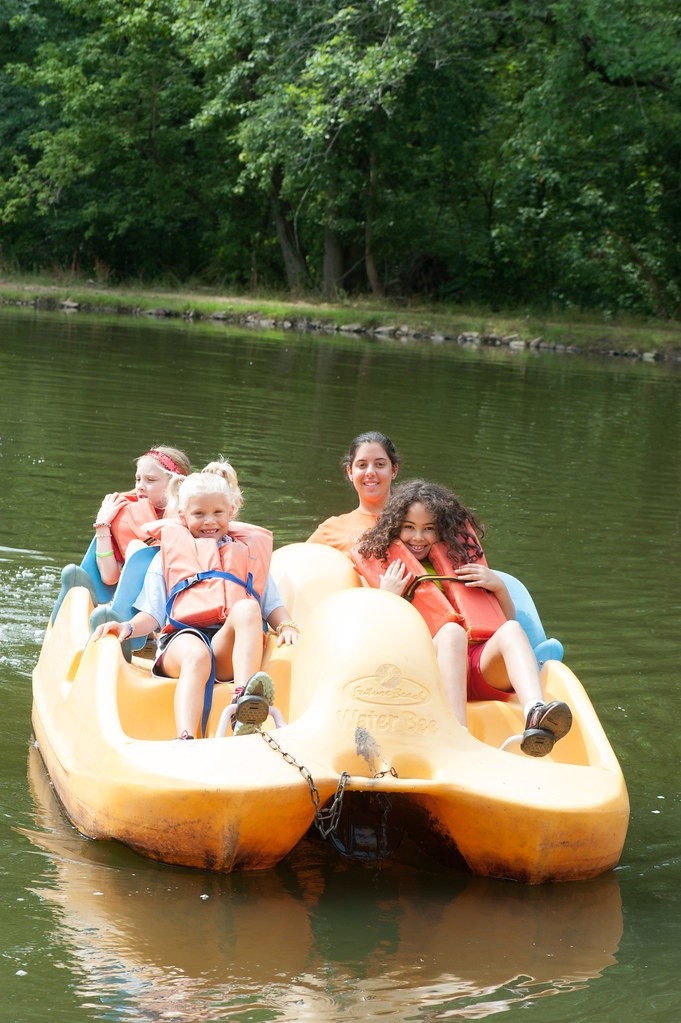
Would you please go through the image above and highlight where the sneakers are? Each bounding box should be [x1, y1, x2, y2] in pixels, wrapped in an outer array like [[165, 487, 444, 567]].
[[520, 701, 572, 758], [175, 729, 194, 740], [231, 670, 276, 737]]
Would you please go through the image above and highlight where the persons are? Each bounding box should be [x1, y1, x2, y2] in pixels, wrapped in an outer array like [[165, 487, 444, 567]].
[[357, 479, 573, 757], [93, 458, 301, 742], [305, 431, 399, 561], [96, 446, 192, 588]]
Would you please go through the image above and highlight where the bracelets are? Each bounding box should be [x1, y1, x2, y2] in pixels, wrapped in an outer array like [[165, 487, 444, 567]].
[[276, 619, 300, 634], [93, 522, 112, 528], [96, 549, 114, 557], [95, 535, 112, 538], [121, 621, 135, 641]]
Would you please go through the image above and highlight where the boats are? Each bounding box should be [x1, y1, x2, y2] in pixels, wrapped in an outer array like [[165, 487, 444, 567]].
[[24, 737, 620, 1013], [30, 542, 629, 886]]
